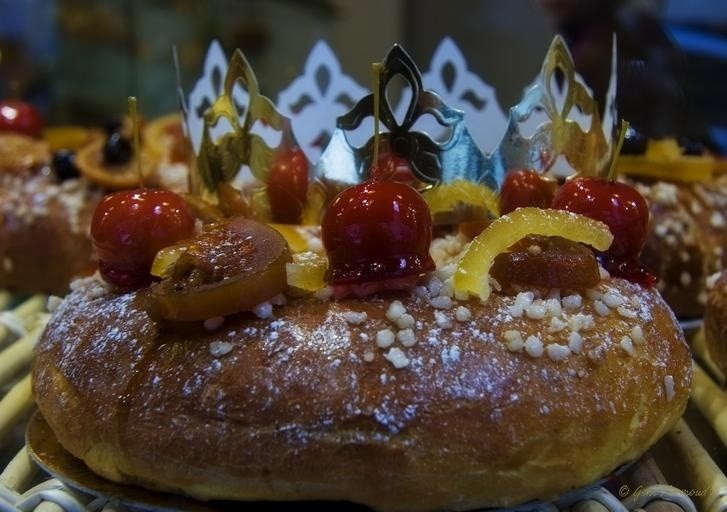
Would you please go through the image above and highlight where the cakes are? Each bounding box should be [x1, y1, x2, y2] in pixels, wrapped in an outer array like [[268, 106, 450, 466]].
[[0, 101, 727, 512]]
